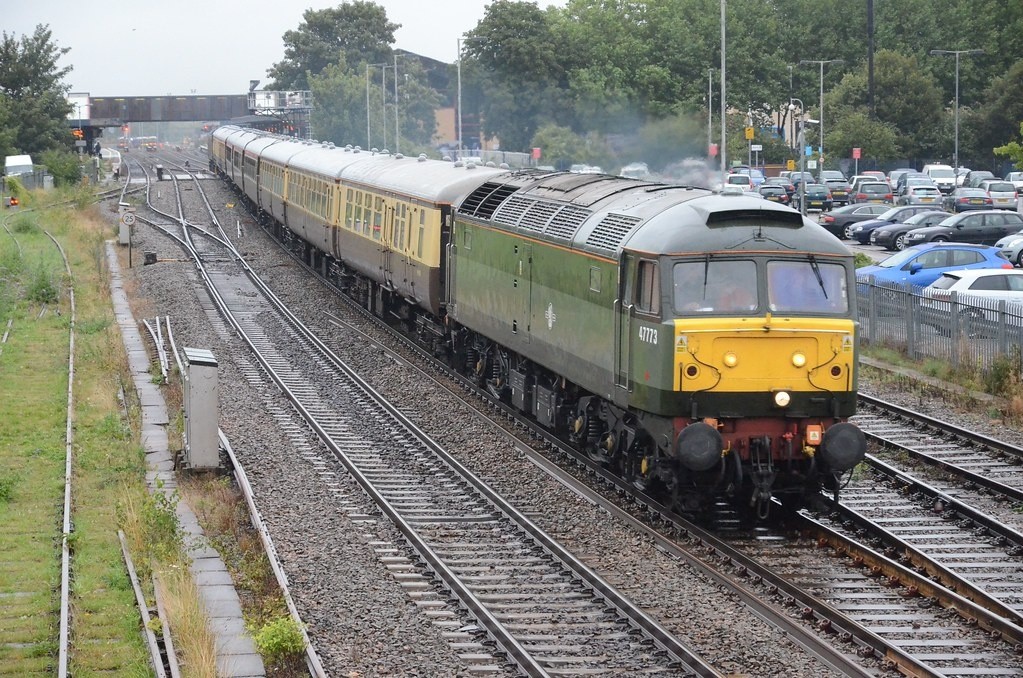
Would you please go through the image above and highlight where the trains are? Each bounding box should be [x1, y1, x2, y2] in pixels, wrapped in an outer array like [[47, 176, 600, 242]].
[[204, 121, 866, 527]]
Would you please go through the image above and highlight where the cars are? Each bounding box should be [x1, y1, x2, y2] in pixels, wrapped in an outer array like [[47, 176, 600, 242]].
[[565, 162, 1023, 343]]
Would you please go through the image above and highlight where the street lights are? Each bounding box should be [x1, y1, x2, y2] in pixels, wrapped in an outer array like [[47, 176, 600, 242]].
[[393, 54, 406, 156], [382, 65, 398, 150], [800, 59, 846, 183], [365, 63, 384, 151], [457, 35, 489, 161], [929, 48, 987, 189]]
